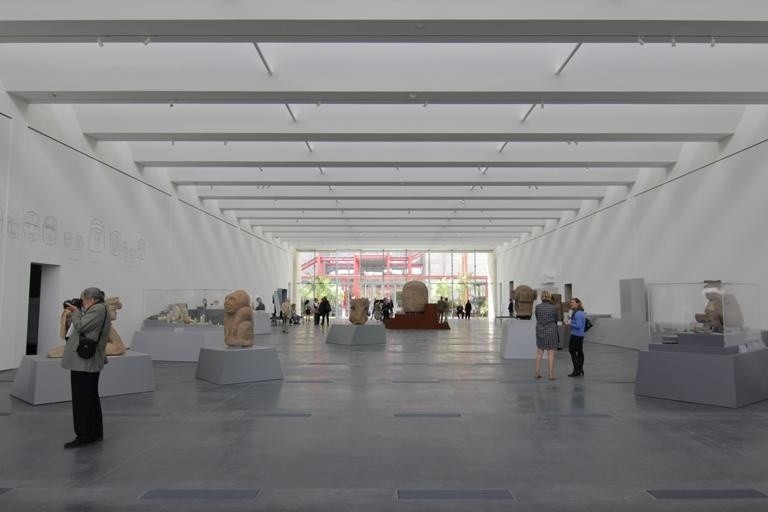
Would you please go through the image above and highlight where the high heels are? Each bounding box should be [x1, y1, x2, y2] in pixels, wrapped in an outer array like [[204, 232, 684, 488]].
[[568, 368, 584, 377]]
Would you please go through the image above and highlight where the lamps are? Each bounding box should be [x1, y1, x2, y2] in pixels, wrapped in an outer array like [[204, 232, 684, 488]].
[[95, 34, 152, 48], [635, 35, 717, 48]]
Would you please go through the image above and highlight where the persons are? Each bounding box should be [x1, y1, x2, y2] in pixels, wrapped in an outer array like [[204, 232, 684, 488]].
[[304, 296, 331, 327], [507, 298, 514, 316], [371, 298, 393, 323], [291, 304, 300, 324], [437, 296, 444, 324], [64, 287, 111, 449], [535, 291, 559, 380], [457, 304, 464, 319], [444, 298, 449, 322], [282, 299, 291, 334], [563, 298, 586, 377], [465, 300, 471, 320]]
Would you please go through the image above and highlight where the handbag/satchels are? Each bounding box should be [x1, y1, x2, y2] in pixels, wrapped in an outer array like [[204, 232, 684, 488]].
[[76, 338, 97, 360], [584, 318, 592, 332]]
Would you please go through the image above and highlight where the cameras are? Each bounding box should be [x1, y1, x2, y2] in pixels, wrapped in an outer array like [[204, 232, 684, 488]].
[[63, 299, 80, 310]]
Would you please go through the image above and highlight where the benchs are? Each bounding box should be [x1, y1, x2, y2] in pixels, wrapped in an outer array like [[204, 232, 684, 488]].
[[270, 315, 305, 325], [451, 311, 476, 318]]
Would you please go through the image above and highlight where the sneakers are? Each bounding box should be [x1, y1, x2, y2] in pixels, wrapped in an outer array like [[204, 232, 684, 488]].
[[64, 436, 103, 449]]
